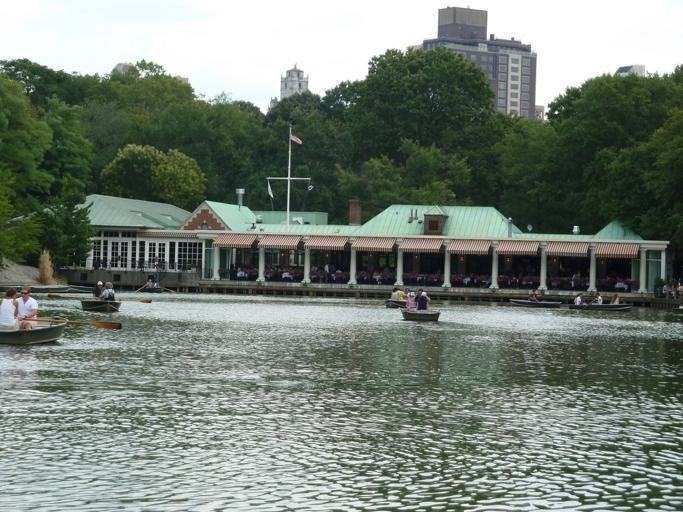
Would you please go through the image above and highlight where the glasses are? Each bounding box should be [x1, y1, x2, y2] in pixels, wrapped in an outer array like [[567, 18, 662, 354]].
[[21, 292, 29, 295]]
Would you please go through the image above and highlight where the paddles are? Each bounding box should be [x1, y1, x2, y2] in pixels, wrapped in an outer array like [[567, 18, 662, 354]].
[[115, 299, 152, 303], [24, 319, 122, 330]]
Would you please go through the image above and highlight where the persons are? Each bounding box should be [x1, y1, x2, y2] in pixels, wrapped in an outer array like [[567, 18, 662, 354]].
[[155, 279, 159, 288], [17, 286, 39, 329], [391, 287, 431, 312], [528, 289, 538, 302], [229, 264, 630, 292], [593, 292, 602, 304], [145, 278, 154, 286], [662, 279, 683, 297], [574, 293, 582, 305], [93, 280, 103, 298], [0, 287, 20, 330], [610, 294, 619, 304], [99, 281, 115, 298]]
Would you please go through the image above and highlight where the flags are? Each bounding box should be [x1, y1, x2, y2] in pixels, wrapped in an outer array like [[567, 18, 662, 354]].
[[267, 180, 274, 198], [291, 135, 303, 144]]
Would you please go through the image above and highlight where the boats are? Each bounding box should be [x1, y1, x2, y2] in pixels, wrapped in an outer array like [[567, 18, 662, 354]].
[[385, 298, 441, 321], [81, 297, 122, 312], [0, 315, 69, 344], [510, 298, 633, 311], [142, 286, 164, 293]]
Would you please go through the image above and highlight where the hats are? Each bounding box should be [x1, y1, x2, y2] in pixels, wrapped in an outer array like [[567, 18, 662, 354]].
[[97, 281, 103, 286]]
